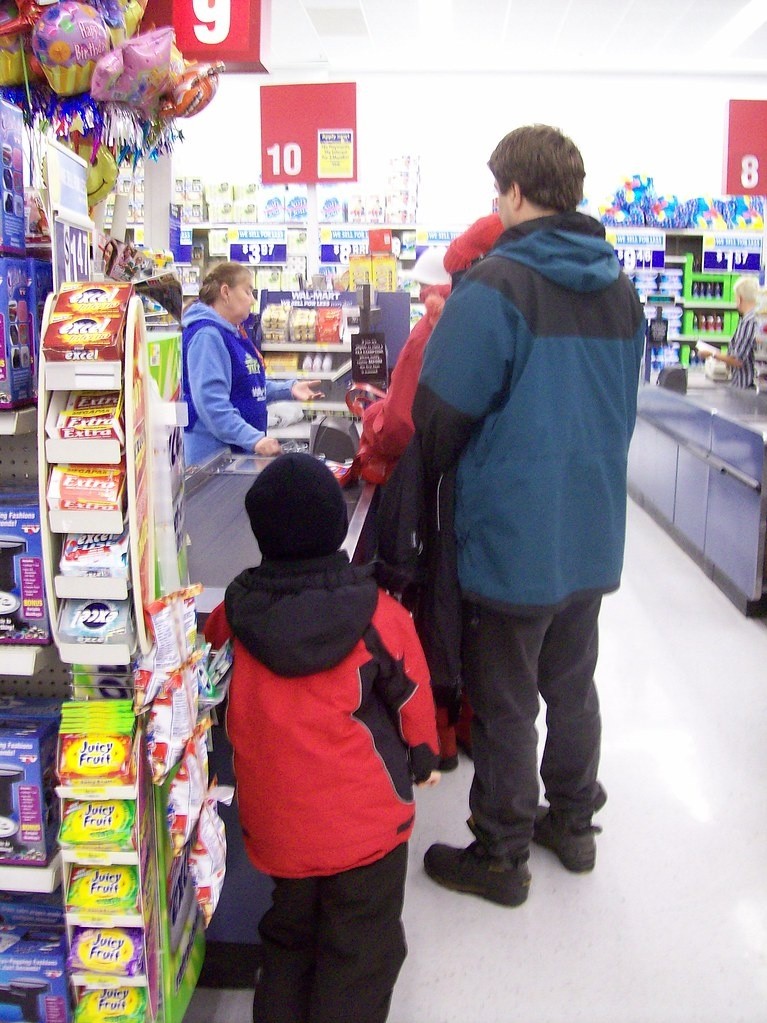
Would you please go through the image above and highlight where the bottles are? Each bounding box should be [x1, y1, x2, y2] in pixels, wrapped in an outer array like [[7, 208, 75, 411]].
[[690, 282, 725, 370]]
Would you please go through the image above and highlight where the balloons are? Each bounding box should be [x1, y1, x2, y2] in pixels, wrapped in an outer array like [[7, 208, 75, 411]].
[[1, 0, 223, 206]]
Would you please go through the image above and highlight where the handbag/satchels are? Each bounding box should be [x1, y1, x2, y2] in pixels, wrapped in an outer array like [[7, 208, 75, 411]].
[[340, 383, 399, 490]]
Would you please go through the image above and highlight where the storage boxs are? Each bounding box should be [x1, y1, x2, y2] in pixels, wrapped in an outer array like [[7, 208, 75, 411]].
[[681, 251, 741, 364], [0, 99, 134, 1021]]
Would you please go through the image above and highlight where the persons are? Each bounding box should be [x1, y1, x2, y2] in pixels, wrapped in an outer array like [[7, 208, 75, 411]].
[[368, 211, 508, 772], [201, 453, 440, 1023], [184, 262, 326, 470], [36, 210, 49, 232], [123, 256, 143, 281], [118, 246, 134, 268], [102, 241, 118, 276], [697, 274, 762, 389], [409, 124, 647, 906]]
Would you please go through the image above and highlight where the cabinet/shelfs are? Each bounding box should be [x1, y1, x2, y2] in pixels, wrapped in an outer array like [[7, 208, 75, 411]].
[[0, 403, 60, 896], [676, 228, 760, 366], [260, 341, 353, 413]]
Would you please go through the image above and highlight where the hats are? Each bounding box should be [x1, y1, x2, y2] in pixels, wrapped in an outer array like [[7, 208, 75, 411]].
[[398, 247, 451, 286], [444, 212, 504, 274], [245, 451, 348, 558]]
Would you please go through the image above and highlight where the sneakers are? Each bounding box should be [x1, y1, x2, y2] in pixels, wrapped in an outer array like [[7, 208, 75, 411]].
[[421, 815, 531, 907], [533, 806, 601, 872]]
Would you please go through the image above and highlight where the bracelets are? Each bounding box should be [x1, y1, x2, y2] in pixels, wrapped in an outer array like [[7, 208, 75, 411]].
[[712, 352, 716, 358]]
